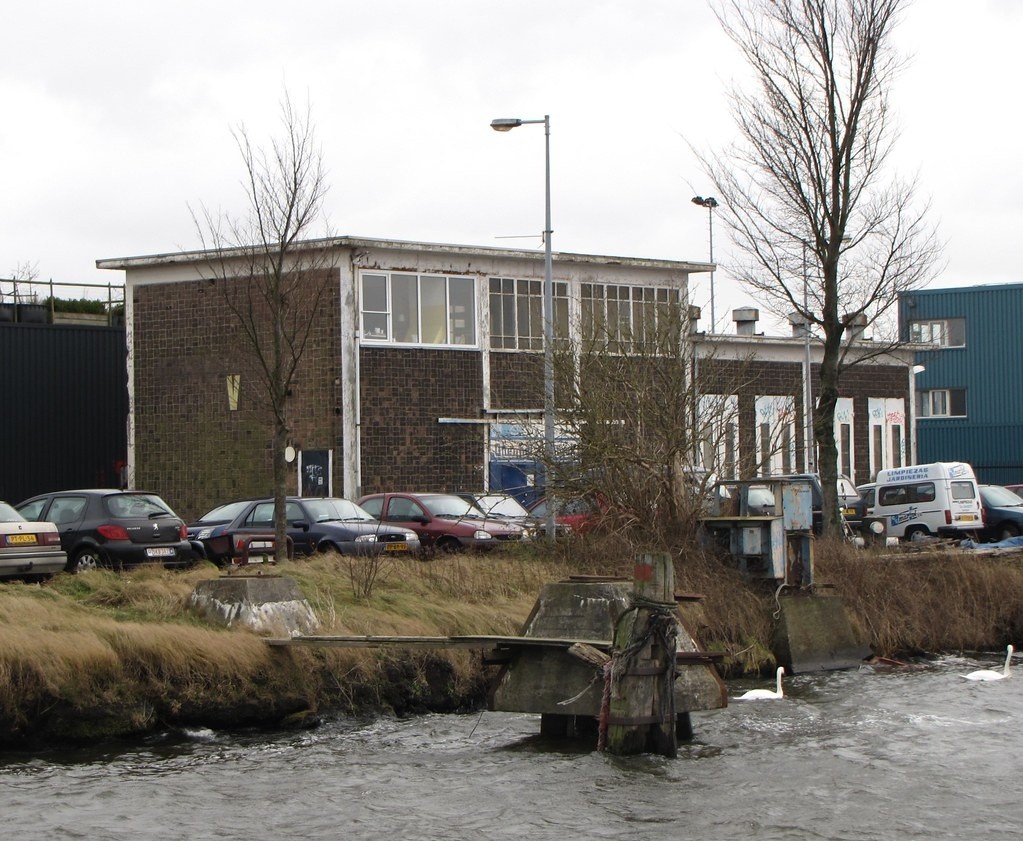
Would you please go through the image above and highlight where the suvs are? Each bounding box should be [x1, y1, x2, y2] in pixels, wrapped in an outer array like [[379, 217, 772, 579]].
[[11, 487, 193, 580]]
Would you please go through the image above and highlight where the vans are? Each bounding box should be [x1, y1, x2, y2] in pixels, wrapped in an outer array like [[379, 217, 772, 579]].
[[656, 456, 1023, 549]]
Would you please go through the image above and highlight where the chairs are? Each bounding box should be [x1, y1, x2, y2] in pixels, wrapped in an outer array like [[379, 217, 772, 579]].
[[59, 509, 75, 523]]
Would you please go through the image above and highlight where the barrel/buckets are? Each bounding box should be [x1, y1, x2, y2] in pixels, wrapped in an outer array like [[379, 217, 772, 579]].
[[886, 536, 899, 547], [851, 537, 864, 546], [870, 521, 884, 533]]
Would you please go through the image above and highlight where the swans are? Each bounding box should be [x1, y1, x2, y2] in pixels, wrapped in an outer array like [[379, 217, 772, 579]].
[[958, 645, 1014, 681], [732, 667, 785, 699]]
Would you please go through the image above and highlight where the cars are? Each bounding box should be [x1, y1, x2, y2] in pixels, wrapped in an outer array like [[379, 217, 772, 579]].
[[354, 491, 529, 556], [449, 493, 575, 546], [183, 495, 423, 567], [528, 489, 632, 535], [0, 499, 68, 583]]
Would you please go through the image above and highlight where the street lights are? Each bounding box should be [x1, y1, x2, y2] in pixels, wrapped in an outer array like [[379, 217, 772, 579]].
[[489, 112, 558, 546], [692, 196, 720, 335], [799, 233, 853, 475]]
